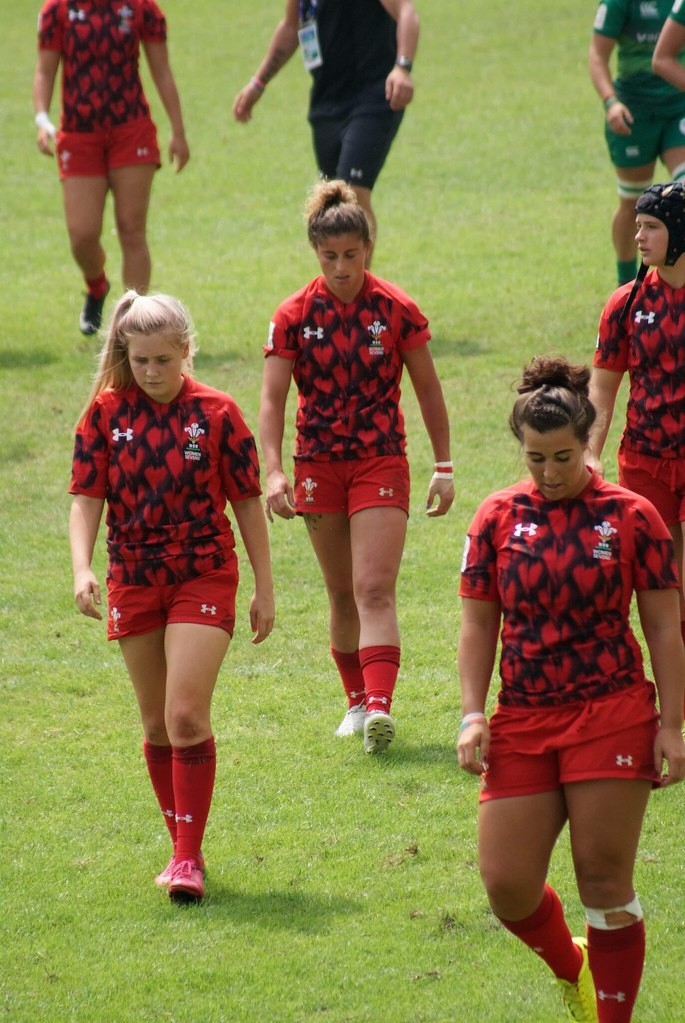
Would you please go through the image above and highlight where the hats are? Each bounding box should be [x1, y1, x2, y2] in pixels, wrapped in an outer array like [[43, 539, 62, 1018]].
[[620, 181, 685, 325]]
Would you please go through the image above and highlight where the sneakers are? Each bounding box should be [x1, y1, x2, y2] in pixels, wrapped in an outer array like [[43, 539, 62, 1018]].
[[153, 856, 175, 888], [168, 859, 204, 900], [80, 281, 111, 333], [556, 936, 598, 1023], [337, 699, 366, 738], [363, 711, 396, 755]]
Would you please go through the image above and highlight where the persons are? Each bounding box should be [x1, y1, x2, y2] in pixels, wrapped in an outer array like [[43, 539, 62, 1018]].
[[32, 0, 190, 334], [67, 290, 275, 898], [232, 0, 420, 269], [456, 357, 685, 1023], [588, 0, 685, 287], [261, 182, 456, 753]]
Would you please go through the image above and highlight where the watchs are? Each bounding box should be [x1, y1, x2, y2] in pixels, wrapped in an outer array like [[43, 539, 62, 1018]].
[[394, 58, 412, 71]]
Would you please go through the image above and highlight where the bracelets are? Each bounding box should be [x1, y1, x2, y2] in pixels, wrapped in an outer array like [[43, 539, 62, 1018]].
[[250, 77, 265, 91], [434, 461, 454, 479], [460, 713, 486, 731], [603, 94, 619, 109]]
